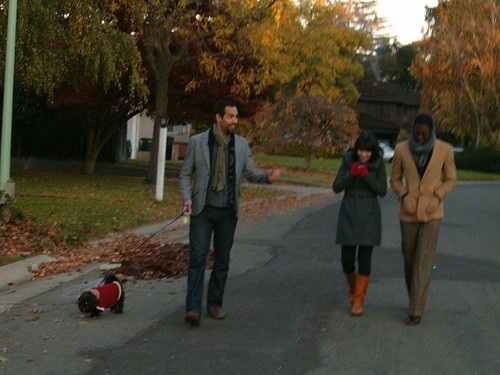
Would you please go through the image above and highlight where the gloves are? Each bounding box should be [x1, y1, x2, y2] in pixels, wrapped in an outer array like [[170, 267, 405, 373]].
[[350, 161, 360, 176], [358, 161, 368, 178]]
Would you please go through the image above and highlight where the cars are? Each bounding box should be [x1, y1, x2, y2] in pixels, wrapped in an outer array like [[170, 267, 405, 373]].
[[378, 142, 395, 163]]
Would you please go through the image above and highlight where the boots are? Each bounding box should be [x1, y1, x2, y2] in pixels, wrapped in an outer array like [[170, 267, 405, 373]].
[[347, 274, 356, 304], [351, 275, 369, 315]]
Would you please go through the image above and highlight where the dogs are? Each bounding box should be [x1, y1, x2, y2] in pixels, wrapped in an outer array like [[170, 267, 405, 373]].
[[74, 271, 127, 321]]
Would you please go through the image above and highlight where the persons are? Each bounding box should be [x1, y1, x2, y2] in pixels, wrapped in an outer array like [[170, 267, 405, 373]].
[[389, 111, 456, 324], [333, 134, 388, 315], [182, 99, 282, 326]]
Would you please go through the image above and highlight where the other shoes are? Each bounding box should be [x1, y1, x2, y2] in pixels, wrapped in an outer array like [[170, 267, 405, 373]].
[[405, 315, 421, 325], [207, 308, 225, 319], [185, 311, 200, 325]]
